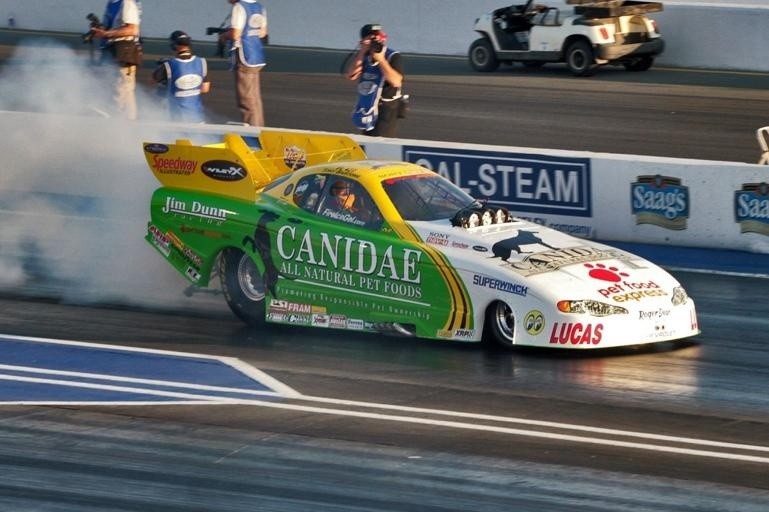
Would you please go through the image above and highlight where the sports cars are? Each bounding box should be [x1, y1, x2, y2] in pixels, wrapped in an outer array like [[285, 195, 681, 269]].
[[140, 127, 704, 354]]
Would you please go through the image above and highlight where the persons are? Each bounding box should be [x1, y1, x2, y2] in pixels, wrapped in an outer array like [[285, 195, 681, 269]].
[[150, 30, 212, 126], [90, 1, 143, 122], [305, 172, 374, 227], [343, 23, 407, 139], [217, 0, 269, 129]]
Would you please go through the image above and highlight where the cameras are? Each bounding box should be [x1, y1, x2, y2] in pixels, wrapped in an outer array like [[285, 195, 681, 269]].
[[206, 28, 231, 58], [81, 13, 107, 42], [368, 38, 383, 52]]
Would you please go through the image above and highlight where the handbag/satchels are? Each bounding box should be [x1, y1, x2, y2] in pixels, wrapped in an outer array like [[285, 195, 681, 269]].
[[105, 40, 138, 66]]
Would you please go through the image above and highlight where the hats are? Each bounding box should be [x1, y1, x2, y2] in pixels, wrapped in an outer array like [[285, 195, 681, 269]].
[[170, 30, 191, 45], [361, 23, 382, 38]]
